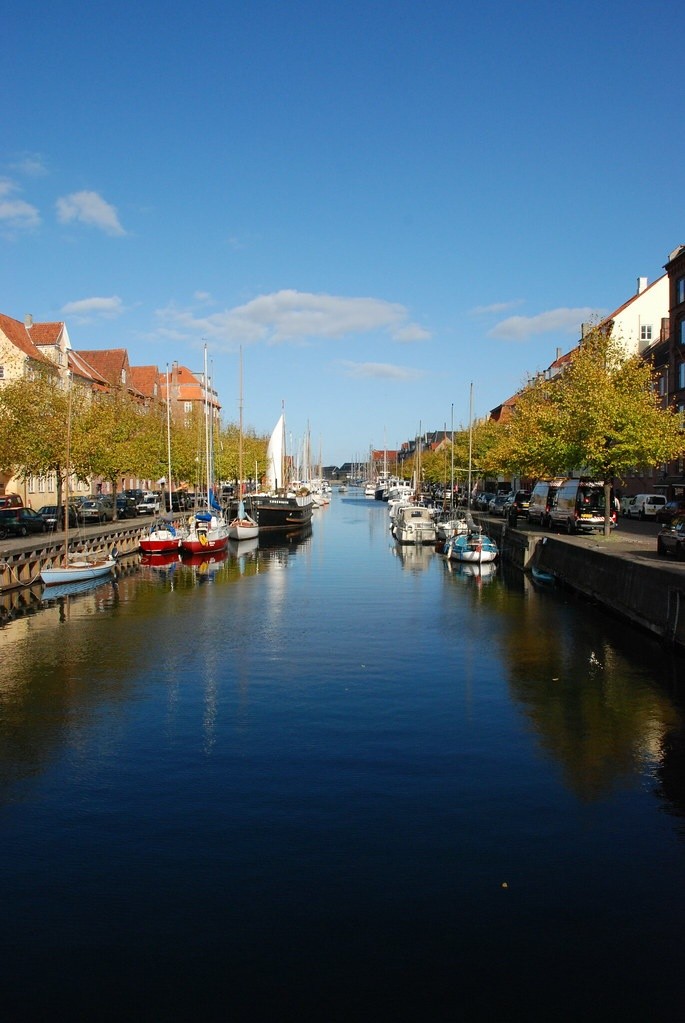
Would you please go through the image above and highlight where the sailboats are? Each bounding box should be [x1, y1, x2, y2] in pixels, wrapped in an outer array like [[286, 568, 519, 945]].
[[226, 343, 259, 542], [138, 365, 190, 555], [170, 340, 231, 556], [441, 551, 497, 607], [39, 360, 117, 586], [435, 383, 498, 565], [183, 545, 229, 590], [140, 549, 186, 593], [41, 570, 118, 624], [226, 536, 260, 575], [224, 399, 473, 544]]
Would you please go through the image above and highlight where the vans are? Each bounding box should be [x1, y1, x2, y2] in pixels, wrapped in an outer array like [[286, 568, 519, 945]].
[[525, 474, 566, 528], [626, 494, 667, 520], [0, 493, 23, 511], [122, 489, 153, 504], [546, 475, 617, 535]]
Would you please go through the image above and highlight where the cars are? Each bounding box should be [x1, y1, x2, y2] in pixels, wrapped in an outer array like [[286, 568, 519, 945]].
[[476, 487, 531, 528], [166, 491, 180, 513], [117, 497, 139, 520], [0, 507, 48, 541], [39, 505, 77, 533], [440, 488, 452, 500], [657, 510, 685, 563], [655, 500, 685, 524], [137, 494, 161, 515], [463, 491, 472, 507], [77, 500, 113, 523]]
